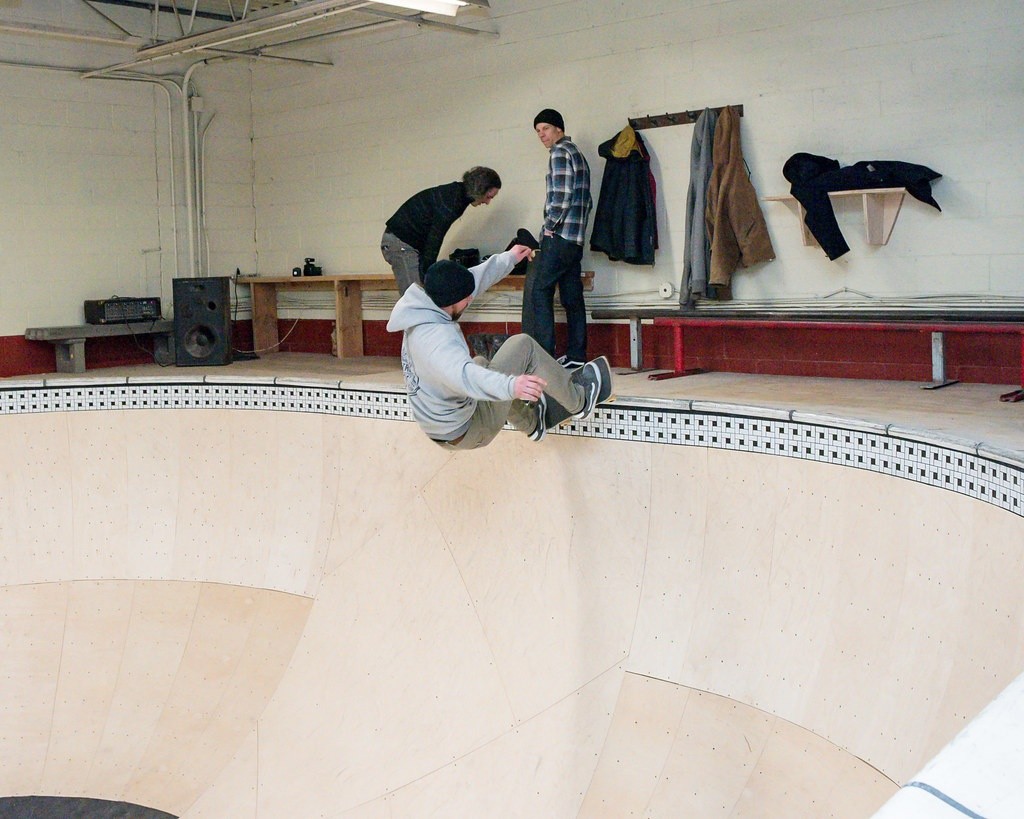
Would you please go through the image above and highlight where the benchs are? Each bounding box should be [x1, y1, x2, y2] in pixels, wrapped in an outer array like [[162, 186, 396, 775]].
[[25, 318, 175, 373], [645, 316, 1024, 403], [590, 307, 1024, 391], [232, 265, 595, 359]]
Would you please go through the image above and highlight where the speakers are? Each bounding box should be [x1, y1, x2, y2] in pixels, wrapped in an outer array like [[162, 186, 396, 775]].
[[171, 276, 233, 368]]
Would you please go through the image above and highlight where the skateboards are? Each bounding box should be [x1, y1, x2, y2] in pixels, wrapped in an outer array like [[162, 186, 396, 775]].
[[541, 355, 616, 431]]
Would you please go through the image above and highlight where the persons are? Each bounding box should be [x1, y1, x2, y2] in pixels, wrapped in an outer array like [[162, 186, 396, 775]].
[[521, 108, 594, 375], [386, 243, 603, 450], [381, 166, 503, 299]]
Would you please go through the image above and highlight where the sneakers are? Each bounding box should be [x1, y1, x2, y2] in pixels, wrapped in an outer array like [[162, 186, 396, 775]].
[[575, 361, 602, 421], [526, 390, 545, 443]]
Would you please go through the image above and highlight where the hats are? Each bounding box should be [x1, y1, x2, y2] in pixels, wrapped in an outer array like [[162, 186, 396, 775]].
[[534, 108, 564, 133], [421, 259, 476, 309]]
